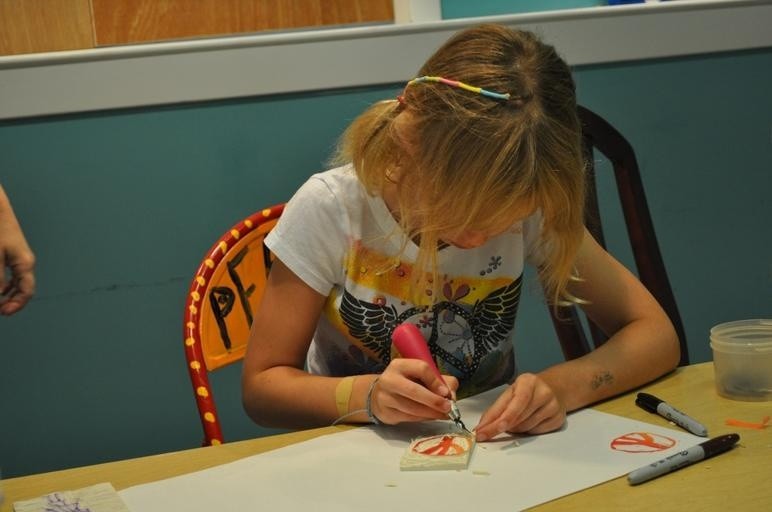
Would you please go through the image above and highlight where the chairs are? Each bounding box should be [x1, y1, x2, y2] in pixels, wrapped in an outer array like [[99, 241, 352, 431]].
[[536, 104, 687, 370], [184, 202, 292, 446]]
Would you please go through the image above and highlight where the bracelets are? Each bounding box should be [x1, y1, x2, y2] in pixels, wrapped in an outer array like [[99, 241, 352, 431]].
[[364, 375, 381, 426]]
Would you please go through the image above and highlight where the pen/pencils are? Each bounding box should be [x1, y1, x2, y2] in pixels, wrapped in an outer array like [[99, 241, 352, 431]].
[[635, 391, 708, 436], [627, 434, 739, 485]]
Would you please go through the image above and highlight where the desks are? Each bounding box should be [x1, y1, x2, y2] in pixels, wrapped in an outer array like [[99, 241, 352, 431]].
[[0, 361, 772, 512]]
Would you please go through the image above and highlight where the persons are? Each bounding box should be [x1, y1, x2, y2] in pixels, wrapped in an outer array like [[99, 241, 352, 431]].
[[1, 184, 38, 316], [241, 22, 682, 444]]
